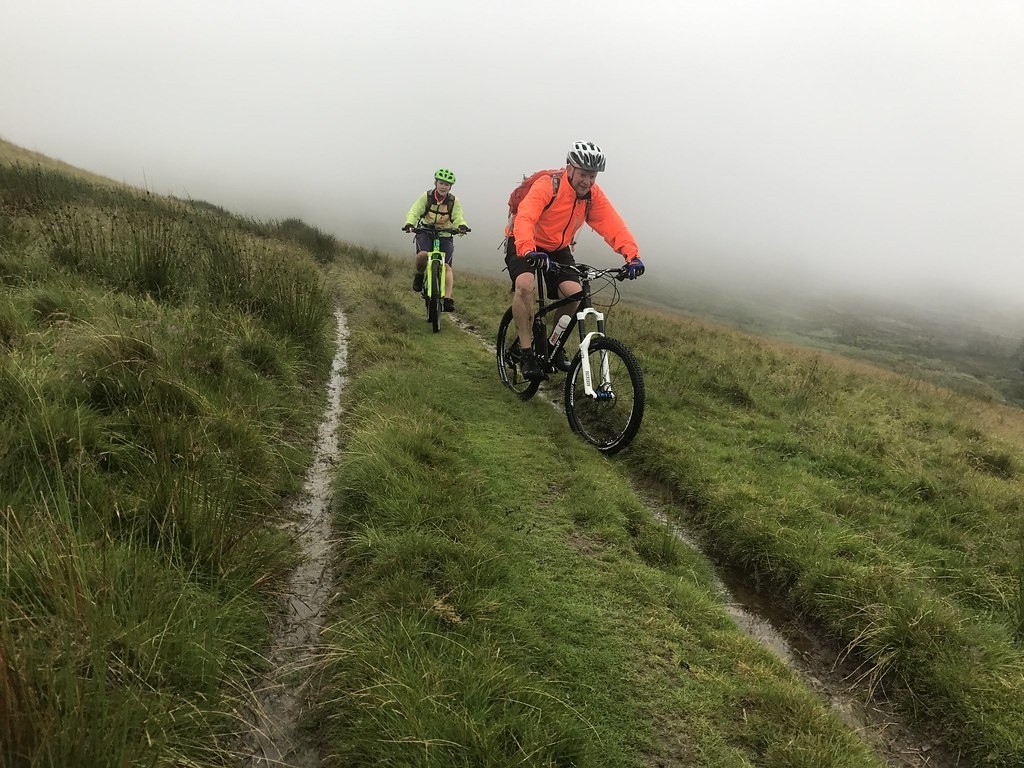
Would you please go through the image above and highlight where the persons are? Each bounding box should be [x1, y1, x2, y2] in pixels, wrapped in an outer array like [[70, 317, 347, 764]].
[[404, 168, 469, 312], [505, 141, 644, 377]]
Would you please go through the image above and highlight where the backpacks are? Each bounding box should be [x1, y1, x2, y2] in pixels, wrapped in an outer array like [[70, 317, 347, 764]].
[[508, 169, 592, 215]]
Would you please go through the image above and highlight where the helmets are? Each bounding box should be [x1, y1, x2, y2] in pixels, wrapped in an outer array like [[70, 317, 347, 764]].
[[434, 168, 456, 184], [567, 140, 606, 171]]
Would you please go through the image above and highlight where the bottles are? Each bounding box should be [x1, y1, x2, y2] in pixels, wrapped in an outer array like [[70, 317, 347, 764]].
[[549, 315, 571, 347]]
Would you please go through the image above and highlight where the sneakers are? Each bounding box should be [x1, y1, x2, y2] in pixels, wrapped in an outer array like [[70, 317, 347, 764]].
[[413, 274, 423, 292], [520, 355, 545, 378], [443, 298, 455, 312], [547, 339, 571, 372]]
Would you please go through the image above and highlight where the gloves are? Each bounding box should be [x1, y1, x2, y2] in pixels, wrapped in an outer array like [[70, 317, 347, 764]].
[[525, 251, 551, 273], [626, 258, 645, 279]]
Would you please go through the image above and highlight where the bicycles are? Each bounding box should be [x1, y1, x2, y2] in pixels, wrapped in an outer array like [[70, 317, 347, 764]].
[[402, 227, 471, 332], [495, 264, 646, 455]]
